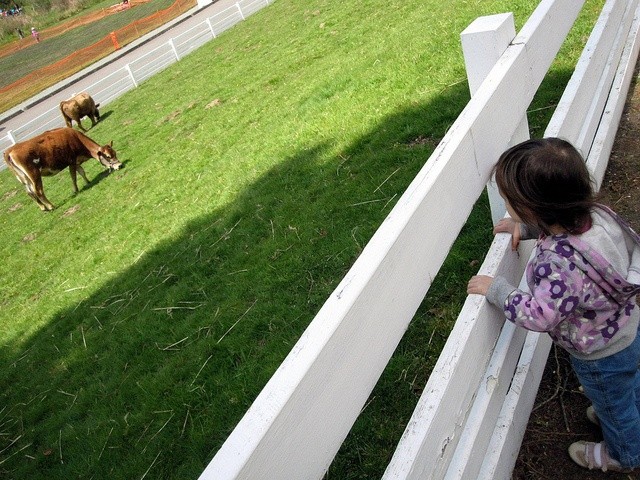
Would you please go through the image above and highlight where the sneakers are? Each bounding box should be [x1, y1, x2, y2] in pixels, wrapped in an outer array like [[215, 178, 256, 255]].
[[587, 404, 601, 425], [569, 441, 634, 473]]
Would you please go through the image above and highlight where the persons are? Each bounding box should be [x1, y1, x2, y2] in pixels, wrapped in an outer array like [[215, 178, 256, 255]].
[[466, 138, 640, 474], [15, 27, 40, 45]]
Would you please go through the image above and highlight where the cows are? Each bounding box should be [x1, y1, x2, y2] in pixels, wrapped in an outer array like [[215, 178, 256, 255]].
[[3, 127, 122, 212], [60, 92, 100, 132]]
